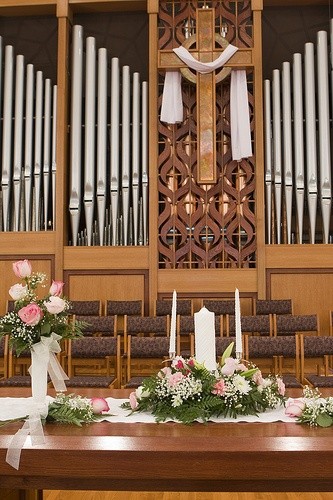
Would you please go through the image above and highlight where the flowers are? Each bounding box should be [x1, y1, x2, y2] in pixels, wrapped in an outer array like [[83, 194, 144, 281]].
[[118, 342, 333, 429], [1, 394, 115, 429], [0, 257, 92, 358]]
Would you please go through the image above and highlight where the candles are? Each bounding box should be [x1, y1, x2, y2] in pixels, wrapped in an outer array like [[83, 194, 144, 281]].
[[235, 288, 243, 357], [168, 288, 178, 357], [194, 305, 216, 370]]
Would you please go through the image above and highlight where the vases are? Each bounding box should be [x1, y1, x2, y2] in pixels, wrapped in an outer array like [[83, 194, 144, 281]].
[[29, 340, 51, 397]]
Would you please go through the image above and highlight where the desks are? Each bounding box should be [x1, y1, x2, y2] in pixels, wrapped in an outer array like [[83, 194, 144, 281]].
[[1, 388, 332, 500]]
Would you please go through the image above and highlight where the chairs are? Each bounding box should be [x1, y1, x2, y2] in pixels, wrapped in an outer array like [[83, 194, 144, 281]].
[[1, 299, 332, 388]]
